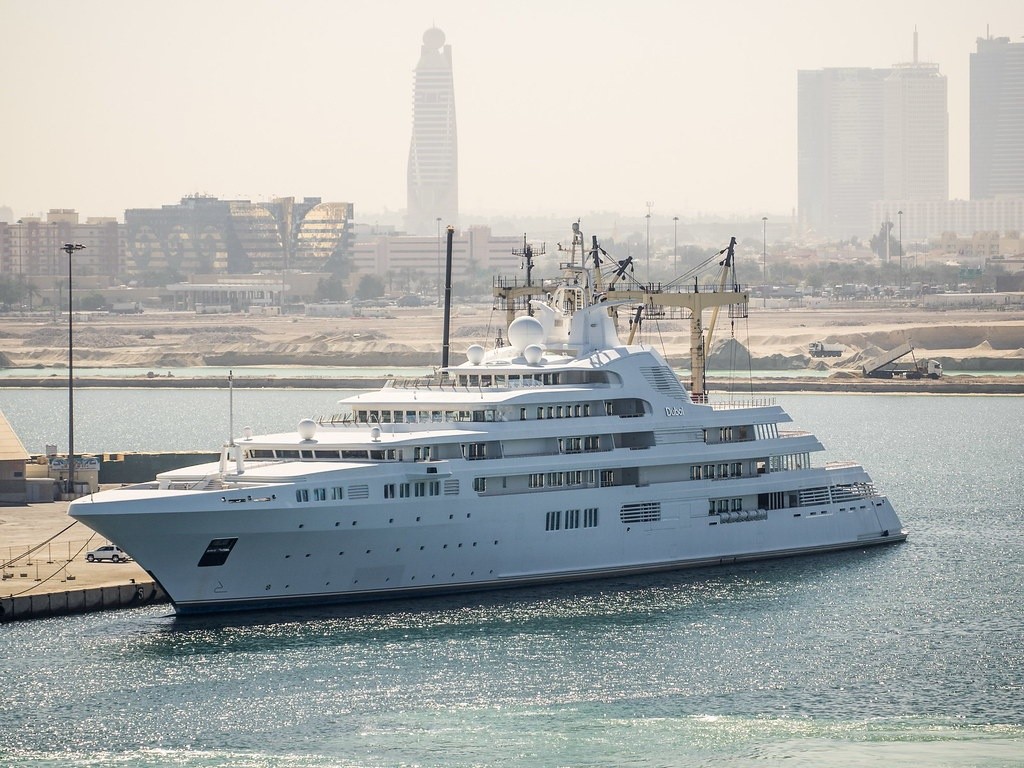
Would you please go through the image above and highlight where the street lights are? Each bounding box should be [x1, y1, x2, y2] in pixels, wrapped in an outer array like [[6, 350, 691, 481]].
[[60, 243, 87, 491], [673, 217, 680, 286], [645, 215, 651, 286], [762, 217, 767, 307], [898, 210, 903, 288], [436, 216, 443, 307]]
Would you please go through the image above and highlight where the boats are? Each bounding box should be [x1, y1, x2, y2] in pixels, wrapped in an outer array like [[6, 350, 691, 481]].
[[66, 307, 909, 621]]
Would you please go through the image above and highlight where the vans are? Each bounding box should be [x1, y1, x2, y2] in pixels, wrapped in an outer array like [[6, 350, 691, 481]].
[[397, 295, 418, 308]]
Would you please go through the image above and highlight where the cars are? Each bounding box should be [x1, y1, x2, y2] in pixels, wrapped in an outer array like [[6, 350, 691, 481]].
[[339, 298, 396, 307], [418, 295, 493, 305], [97, 306, 109, 311], [747, 284, 994, 298]]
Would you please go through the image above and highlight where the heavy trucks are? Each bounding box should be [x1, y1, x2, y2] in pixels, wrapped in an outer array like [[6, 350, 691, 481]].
[[808, 341, 845, 358], [110, 302, 145, 315], [862, 341, 943, 379]]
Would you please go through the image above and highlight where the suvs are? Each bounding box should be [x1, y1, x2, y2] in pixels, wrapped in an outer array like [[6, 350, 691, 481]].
[[85, 544, 130, 563]]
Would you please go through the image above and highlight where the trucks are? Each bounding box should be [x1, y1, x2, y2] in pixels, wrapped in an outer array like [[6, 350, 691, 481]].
[[320, 298, 338, 305]]
